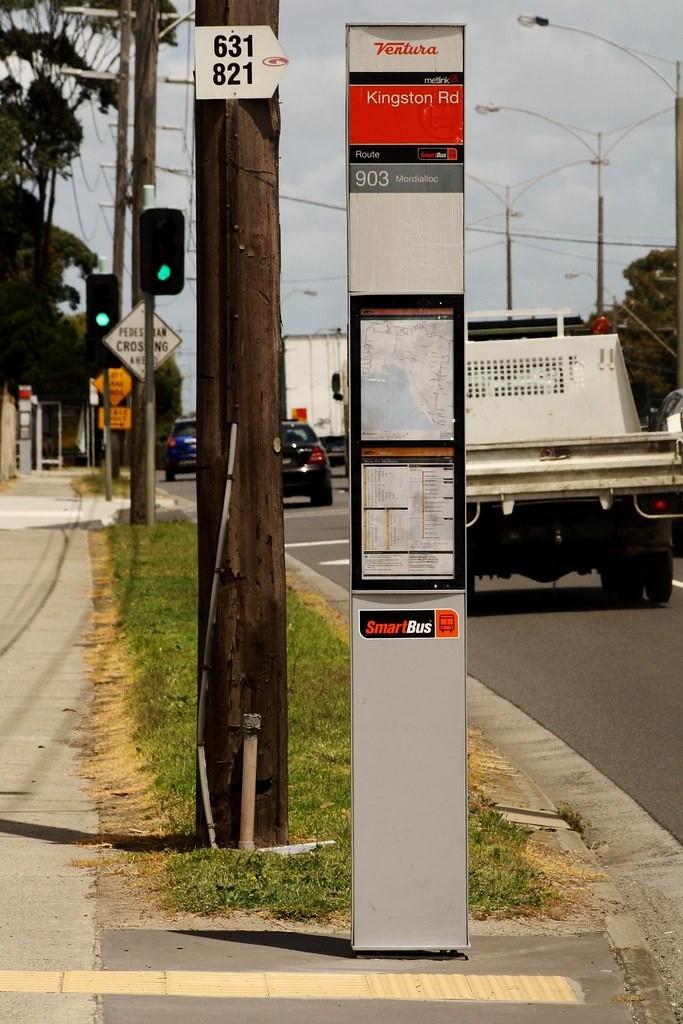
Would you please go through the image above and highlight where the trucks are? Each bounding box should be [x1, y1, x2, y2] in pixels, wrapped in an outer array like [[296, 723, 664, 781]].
[[465, 307, 683, 616], [283, 334, 347, 466]]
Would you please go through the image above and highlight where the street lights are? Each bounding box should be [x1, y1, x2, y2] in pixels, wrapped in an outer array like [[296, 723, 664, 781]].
[[566, 272, 618, 334], [464, 158, 610, 321], [475, 104, 675, 318], [518, 14, 682, 388]]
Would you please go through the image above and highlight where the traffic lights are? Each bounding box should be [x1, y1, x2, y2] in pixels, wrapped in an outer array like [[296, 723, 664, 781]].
[[85, 273, 119, 336], [140, 208, 185, 295]]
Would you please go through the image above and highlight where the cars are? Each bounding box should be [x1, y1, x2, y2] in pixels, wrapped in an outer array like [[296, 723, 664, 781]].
[[650, 388, 683, 559], [280, 422, 333, 507], [159, 418, 197, 482]]
